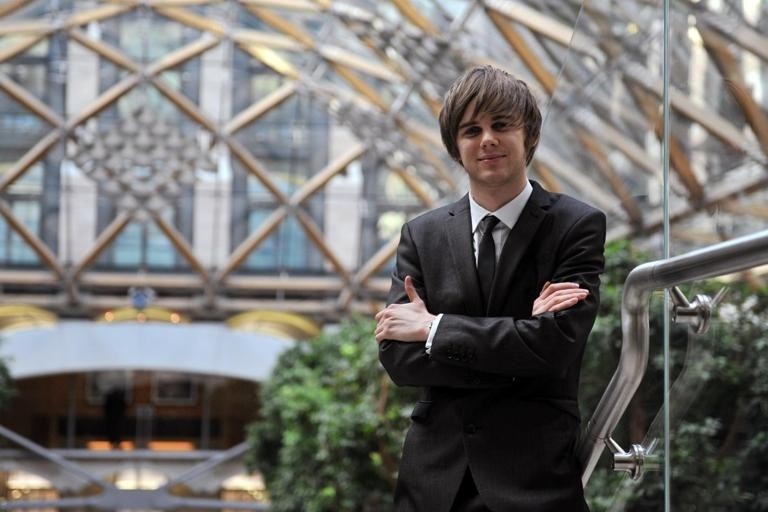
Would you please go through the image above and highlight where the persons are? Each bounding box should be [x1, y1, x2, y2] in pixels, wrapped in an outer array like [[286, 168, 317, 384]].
[[373, 63, 607, 512]]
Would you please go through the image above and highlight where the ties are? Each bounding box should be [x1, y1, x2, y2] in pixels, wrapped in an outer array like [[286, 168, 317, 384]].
[[477, 216, 500, 302]]
